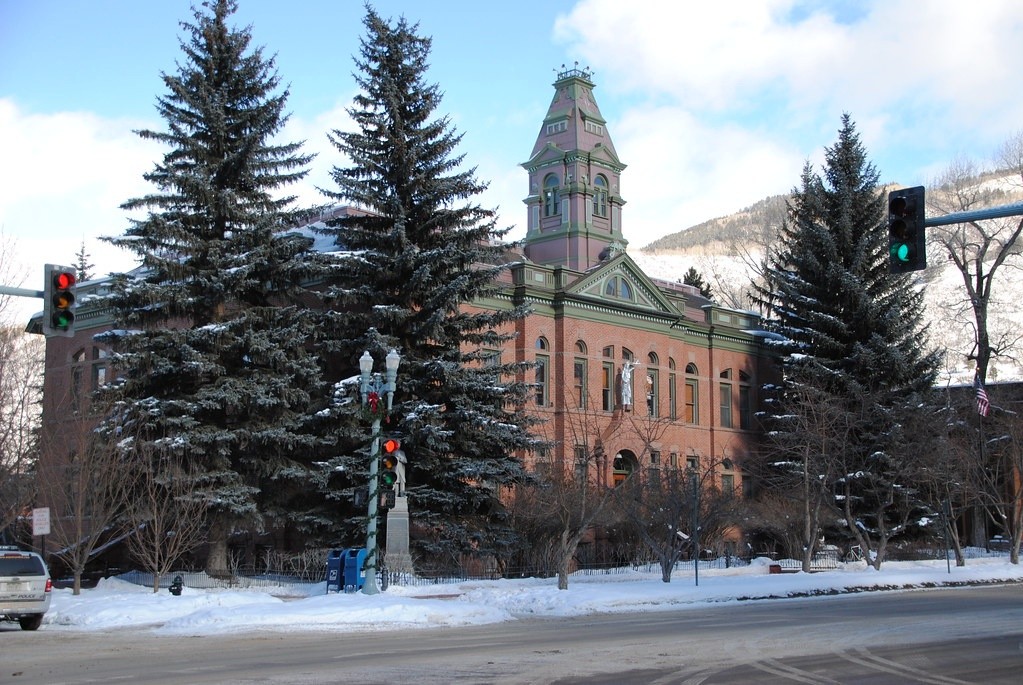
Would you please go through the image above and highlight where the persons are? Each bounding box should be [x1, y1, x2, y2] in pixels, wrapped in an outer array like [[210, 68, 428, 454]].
[[621, 360, 635, 405]]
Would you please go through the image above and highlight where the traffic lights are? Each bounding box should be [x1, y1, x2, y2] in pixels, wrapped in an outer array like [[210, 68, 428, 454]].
[[887, 186, 928, 272], [378, 435, 397, 490], [43, 263, 77, 339]]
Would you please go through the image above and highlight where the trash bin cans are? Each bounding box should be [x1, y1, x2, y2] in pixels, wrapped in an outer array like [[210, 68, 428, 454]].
[[325, 547, 368, 595]]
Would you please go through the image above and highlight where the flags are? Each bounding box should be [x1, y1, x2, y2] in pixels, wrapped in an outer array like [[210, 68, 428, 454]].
[[976, 373, 991, 418]]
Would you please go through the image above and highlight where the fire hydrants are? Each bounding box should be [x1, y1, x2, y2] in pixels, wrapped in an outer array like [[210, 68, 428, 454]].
[[168, 575, 183, 595]]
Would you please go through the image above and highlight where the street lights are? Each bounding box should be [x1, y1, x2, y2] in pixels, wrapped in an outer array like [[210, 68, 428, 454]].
[[356, 348, 400, 597]]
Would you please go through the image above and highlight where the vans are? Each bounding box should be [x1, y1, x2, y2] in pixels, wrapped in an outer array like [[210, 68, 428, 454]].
[[0, 546, 53, 630]]
